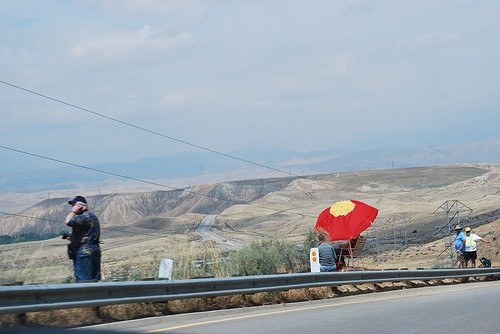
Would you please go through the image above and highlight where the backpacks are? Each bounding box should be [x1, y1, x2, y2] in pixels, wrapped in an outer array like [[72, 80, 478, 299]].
[[482, 257, 491, 268]]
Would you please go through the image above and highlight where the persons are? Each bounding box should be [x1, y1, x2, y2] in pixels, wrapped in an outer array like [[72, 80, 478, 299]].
[[61, 196, 101, 281], [318, 234, 337, 272], [452, 225, 489, 281], [335, 235, 363, 269]]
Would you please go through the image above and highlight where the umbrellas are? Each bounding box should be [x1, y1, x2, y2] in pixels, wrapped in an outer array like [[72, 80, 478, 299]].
[[314, 200, 379, 240]]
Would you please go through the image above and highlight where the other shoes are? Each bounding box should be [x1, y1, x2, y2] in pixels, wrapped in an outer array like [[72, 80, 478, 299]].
[[453, 279, 460, 283], [473, 279, 478, 281]]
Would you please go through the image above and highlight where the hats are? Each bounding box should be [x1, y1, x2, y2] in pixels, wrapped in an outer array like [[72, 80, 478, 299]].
[[465, 227, 472, 231], [454, 225, 462, 229], [68, 195, 87, 204]]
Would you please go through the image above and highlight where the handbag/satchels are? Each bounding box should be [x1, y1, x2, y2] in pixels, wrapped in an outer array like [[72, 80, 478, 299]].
[[68, 243, 73, 259]]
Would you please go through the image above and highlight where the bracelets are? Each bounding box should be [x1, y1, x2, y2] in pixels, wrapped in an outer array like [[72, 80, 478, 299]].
[[69, 210, 77, 214]]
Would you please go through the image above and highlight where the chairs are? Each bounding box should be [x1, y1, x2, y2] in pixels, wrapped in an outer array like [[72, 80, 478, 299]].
[[437, 237, 465, 267], [335, 234, 367, 270]]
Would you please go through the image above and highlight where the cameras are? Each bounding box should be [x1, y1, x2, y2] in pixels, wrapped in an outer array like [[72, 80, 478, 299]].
[[62, 231, 73, 240]]
